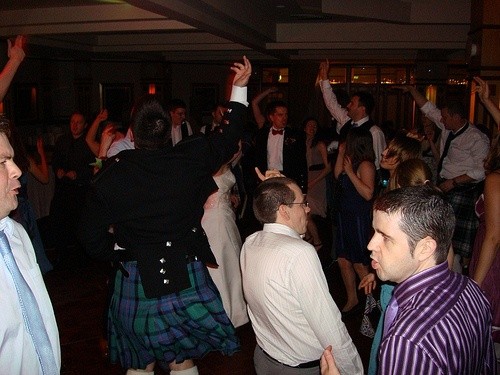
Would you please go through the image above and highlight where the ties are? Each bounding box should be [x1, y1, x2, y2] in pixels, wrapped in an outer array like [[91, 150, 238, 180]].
[[436, 123, 469, 176], [0, 229, 60, 375], [352, 123, 358, 127]]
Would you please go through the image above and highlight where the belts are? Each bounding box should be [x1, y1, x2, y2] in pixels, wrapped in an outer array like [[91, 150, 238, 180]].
[[257, 342, 321, 368]]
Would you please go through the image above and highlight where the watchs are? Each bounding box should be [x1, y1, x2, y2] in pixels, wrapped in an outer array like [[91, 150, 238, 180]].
[[452, 178, 458, 187]]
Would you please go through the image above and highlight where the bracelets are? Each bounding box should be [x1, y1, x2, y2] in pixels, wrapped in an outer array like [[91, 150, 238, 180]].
[[411, 91, 418, 96]]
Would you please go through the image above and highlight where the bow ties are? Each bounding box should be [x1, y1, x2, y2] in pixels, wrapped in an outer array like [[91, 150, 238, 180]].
[[272, 129, 283, 136]]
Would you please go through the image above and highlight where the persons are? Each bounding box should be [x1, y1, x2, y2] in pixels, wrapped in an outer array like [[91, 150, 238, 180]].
[[0, 35, 500, 375]]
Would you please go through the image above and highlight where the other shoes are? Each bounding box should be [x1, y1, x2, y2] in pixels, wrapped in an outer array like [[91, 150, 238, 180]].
[[338, 302, 363, 314]]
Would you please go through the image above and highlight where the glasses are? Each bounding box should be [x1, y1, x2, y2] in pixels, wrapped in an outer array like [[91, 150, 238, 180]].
[[279, 201, 308, 208]]
